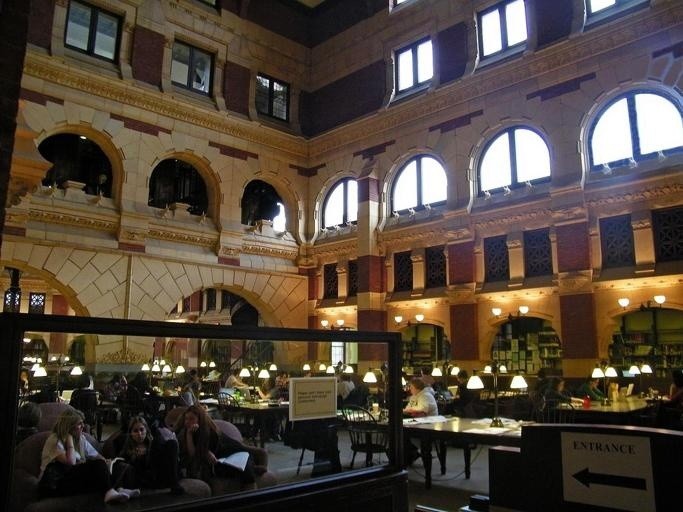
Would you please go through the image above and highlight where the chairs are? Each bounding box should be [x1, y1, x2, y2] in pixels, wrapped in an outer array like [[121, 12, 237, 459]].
[[116, 380, 154, 436], [67, 388, 102, 441]]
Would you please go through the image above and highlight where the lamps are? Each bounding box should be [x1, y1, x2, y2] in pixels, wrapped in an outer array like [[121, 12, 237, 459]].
[[28, 356, 83, 402], [464, 361, 530, 427], [196, 353, 278, 405], [139, 356, 185, 384], [589, 358, 655, 406], [431, 359, 459, 391], [298, 359, 406, 410]]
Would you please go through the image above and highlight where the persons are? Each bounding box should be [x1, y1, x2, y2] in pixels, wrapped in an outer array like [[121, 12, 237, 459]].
[[12, 356, 682, 509]]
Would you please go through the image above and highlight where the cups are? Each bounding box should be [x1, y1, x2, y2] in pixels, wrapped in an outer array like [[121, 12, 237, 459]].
[[372, 402, 378, 413]]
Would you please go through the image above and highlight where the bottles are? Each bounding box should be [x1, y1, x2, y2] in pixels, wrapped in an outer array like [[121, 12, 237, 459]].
[[235, 389, 240, 401], [582, 392, 590, 407]]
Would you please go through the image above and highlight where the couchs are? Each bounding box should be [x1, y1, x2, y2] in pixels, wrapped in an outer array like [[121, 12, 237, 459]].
[[12, 398, 278, 512]]
